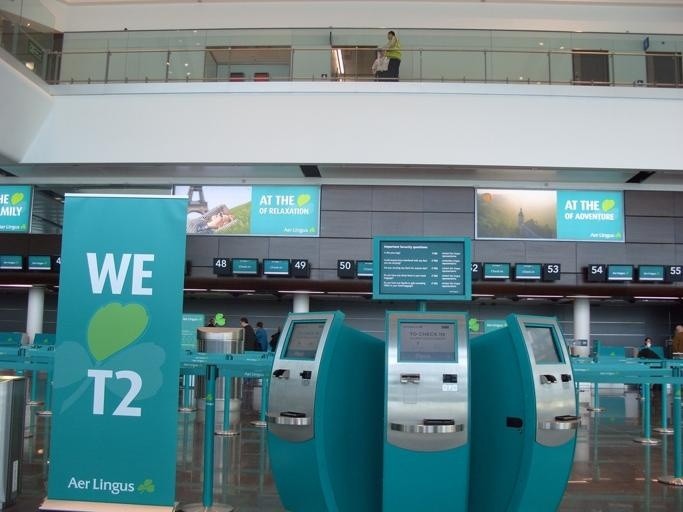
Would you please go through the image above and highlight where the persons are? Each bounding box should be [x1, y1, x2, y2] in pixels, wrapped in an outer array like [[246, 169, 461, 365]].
[[269, 325, 281, 352], [371, 31, 401, 82], [239, 317, 267, 352], [207, 318, 216, 326], [185, 204, 241, 234], [671, 325, 683, 358], [643, 336, 653, 346]]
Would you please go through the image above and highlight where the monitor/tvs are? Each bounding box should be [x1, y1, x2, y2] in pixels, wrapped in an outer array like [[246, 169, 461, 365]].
[[372, 237, 472, 301], [263, 259, 289, 275], [356, 260, 373, 276], [27, 255, 51, 270], [514, 263, 542, 279], [0, 255, 23, 270], [231, 258, 258, 274], [284, 322, 325, 358], [525, 326, 562, 362], [400, 323, 454, 362], [483, 262, 510, 279], [638, 265, 664, 280], [607, 264, 634, 281]]
